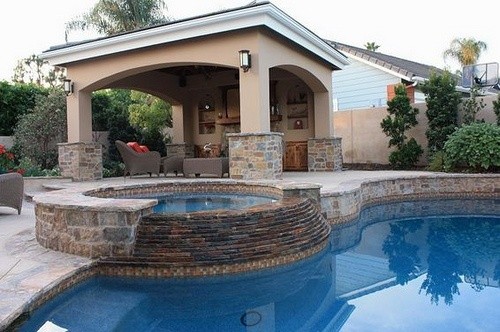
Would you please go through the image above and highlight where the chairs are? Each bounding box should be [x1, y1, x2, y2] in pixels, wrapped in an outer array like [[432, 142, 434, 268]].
[[0, 172, 23, 215], [115, 140, 161, 178]]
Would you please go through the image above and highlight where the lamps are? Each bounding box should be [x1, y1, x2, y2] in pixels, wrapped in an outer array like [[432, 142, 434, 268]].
[[63, 79, 74, 96], [238, 49, 251, 73]]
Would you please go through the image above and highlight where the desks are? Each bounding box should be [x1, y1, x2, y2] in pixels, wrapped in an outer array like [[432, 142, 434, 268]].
[[165, 143, 199, 177], [183, 157, 229, 178]]
